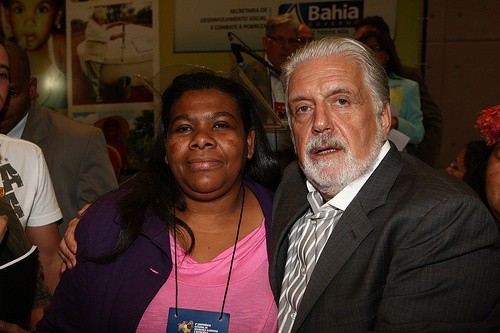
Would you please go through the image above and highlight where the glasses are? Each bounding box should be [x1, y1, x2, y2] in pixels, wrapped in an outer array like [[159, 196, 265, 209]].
[[295, 37, 314, 44], [267, 33, 297, 45], [369, 45, 384, 52]]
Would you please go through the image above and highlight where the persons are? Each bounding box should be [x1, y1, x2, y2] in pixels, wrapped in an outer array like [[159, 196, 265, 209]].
[[0, 43, 63, 297], [29, 68, 281, 333], [227, 12, 317, 195], [0, 194, 52, 333], [0, 39, 120, 242], [82, 6, 128, 103], [351, 16, 441, 163], [445, 140, 500, 233], [0, 0, 68, 110], [55, 35, 500, 333]]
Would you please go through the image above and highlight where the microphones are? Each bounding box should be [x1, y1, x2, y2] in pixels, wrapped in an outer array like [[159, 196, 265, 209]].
[[227, 32, 245, 67]]
